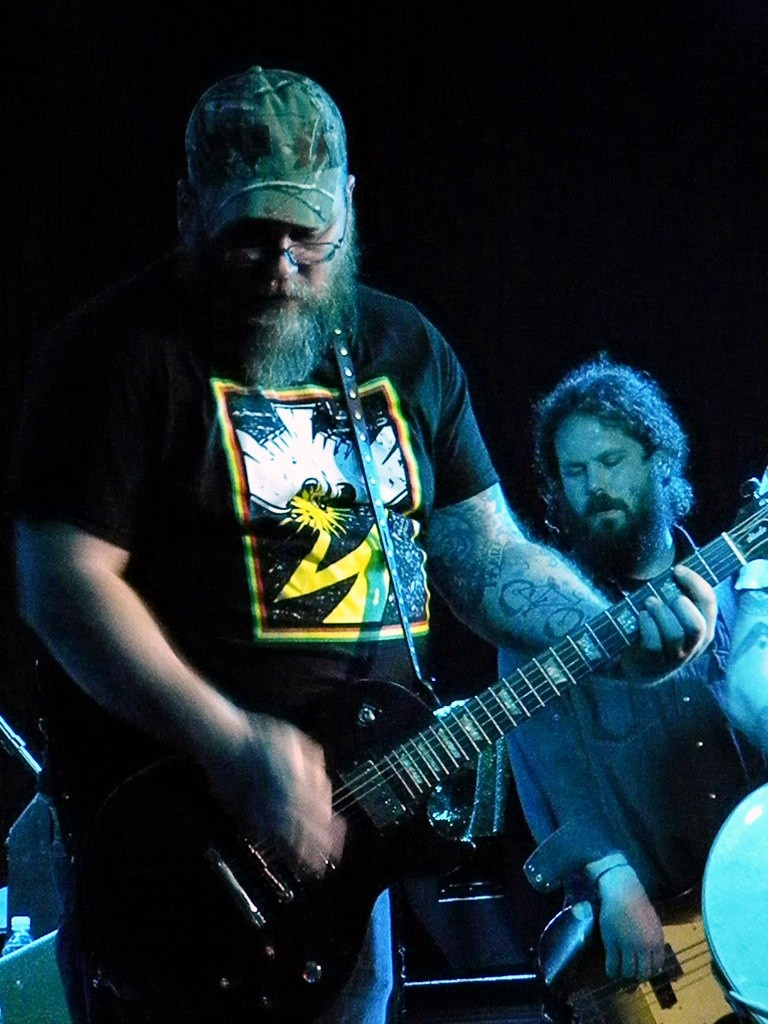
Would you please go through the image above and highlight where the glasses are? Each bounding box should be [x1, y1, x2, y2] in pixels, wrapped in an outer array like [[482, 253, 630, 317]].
[[204, 190, 350, 269]]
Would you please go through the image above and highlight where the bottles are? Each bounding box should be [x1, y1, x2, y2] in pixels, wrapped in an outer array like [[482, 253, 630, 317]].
[[2, 916, 34, 956]]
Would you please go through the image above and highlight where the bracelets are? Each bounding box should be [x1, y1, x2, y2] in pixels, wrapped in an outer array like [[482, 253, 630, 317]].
[[606, 649, 624, 680], [585, 854, 629, 884]]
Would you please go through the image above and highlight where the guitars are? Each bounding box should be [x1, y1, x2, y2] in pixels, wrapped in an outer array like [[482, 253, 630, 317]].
[[535, 876, 735, 1024], [77, 458, 768, 1024]]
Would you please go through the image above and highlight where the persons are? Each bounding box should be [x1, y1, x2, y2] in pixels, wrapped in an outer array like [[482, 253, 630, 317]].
[[13, 61, 719, 1024], [725, 456, 768, 749], [497, 364, 752, 984]]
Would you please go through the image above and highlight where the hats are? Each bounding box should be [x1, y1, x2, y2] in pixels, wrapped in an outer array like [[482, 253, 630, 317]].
[[180, 66, 348, 243]]
